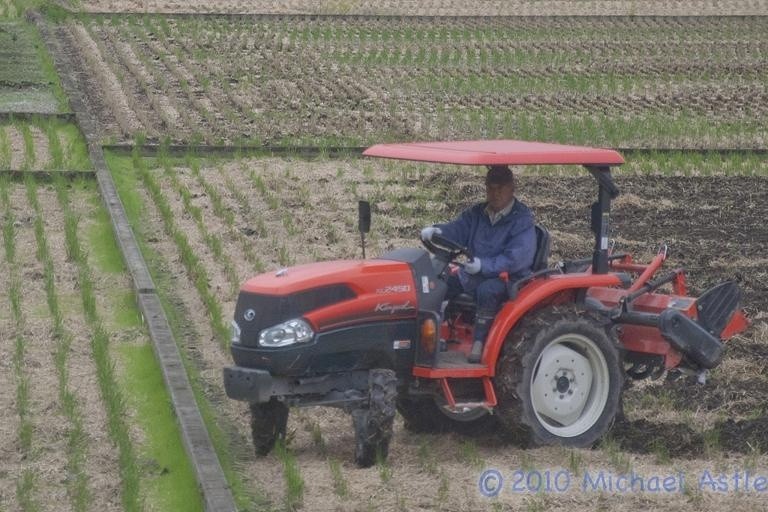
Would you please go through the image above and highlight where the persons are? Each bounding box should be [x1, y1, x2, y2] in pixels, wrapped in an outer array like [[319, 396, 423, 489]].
[[420, 164, 539, 365]]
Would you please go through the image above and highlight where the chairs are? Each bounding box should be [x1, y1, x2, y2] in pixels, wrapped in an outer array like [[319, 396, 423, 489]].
[[449, 223, 552, 323]]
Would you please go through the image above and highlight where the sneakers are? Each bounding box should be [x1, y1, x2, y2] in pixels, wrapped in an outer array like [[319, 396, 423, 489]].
[[465, 338, 485, 363]]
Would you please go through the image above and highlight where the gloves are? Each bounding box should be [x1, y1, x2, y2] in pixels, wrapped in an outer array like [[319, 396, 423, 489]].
[[464, 255, 482, 275], [420, 227, 442, 243]]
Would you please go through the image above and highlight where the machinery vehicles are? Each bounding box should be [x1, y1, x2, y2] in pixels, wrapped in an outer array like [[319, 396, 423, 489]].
[[223, 138, 748, 467]]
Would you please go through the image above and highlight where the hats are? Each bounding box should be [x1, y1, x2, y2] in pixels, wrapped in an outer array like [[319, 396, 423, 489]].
[[484, 166, 514, 185]]
